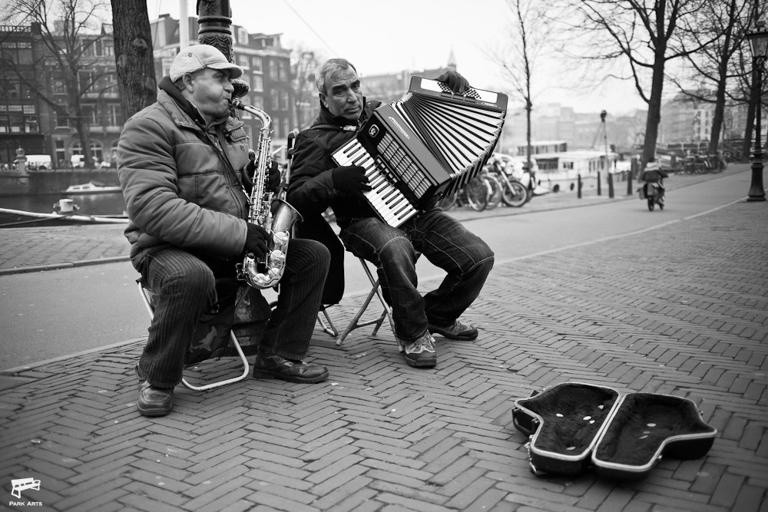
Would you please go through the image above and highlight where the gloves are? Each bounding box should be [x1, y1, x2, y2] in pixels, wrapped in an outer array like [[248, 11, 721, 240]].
[[243, 221, 274, 257], [438, 69, 470, 93], [332, 165, 371, 194], [247, 150, 281, 191]]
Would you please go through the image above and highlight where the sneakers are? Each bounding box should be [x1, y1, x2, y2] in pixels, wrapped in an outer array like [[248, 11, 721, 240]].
[[432, 316, 479, 340], [401, 329, 441, 371]]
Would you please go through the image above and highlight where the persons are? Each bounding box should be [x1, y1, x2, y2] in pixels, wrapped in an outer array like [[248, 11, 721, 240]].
[[641, 157, 667, 208], [285, 57, 495, 367], [115, 41, 334, 418]]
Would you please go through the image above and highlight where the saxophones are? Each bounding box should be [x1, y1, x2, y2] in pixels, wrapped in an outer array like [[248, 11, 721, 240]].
[[228, 96, 304, 292]]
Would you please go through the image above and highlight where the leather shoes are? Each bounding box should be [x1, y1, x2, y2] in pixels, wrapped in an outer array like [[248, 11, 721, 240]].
[[252, 353, 330, 385], [133, 372, 176, 419]]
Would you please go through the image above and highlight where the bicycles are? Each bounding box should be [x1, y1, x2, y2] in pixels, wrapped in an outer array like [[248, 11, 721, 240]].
[[436, 151, 540, 211], [672, 149, 726, 174]]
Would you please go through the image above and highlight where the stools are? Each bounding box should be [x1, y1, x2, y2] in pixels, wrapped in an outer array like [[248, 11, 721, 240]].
[[334, 243, 423, 353], [134, 268, 254, 392]]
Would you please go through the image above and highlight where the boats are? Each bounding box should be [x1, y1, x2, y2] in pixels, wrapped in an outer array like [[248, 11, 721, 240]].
[[513, 106, 672, 198]]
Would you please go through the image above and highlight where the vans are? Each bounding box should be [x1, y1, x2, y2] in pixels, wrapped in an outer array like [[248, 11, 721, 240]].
[[2, 152, 53, 170], [720, 137, 767, 160], [69, 153, 112, 169]]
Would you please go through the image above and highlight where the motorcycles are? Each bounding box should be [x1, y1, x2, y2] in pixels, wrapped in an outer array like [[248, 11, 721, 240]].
[[643, 173, 667, 211]]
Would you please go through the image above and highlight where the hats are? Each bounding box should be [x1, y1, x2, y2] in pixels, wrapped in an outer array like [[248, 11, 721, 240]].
[[169, 43, 245, 83]]
